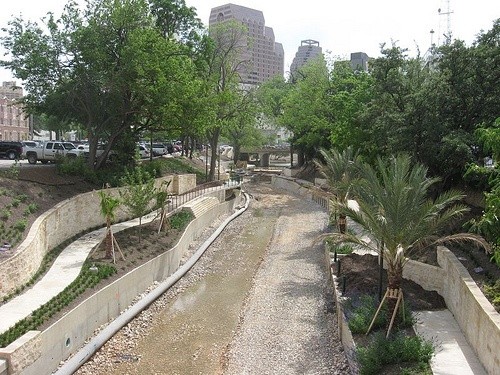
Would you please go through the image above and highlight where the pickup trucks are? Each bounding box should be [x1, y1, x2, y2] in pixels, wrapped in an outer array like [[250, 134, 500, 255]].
[[23, 142, 87, 164], [0, 142, 22, 159]]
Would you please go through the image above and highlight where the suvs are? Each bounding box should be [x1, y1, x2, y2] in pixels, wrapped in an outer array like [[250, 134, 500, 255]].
[[94, 144, 121, 161]]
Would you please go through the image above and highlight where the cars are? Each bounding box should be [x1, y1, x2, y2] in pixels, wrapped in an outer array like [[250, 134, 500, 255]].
[[137, 141, 182, 158]]
